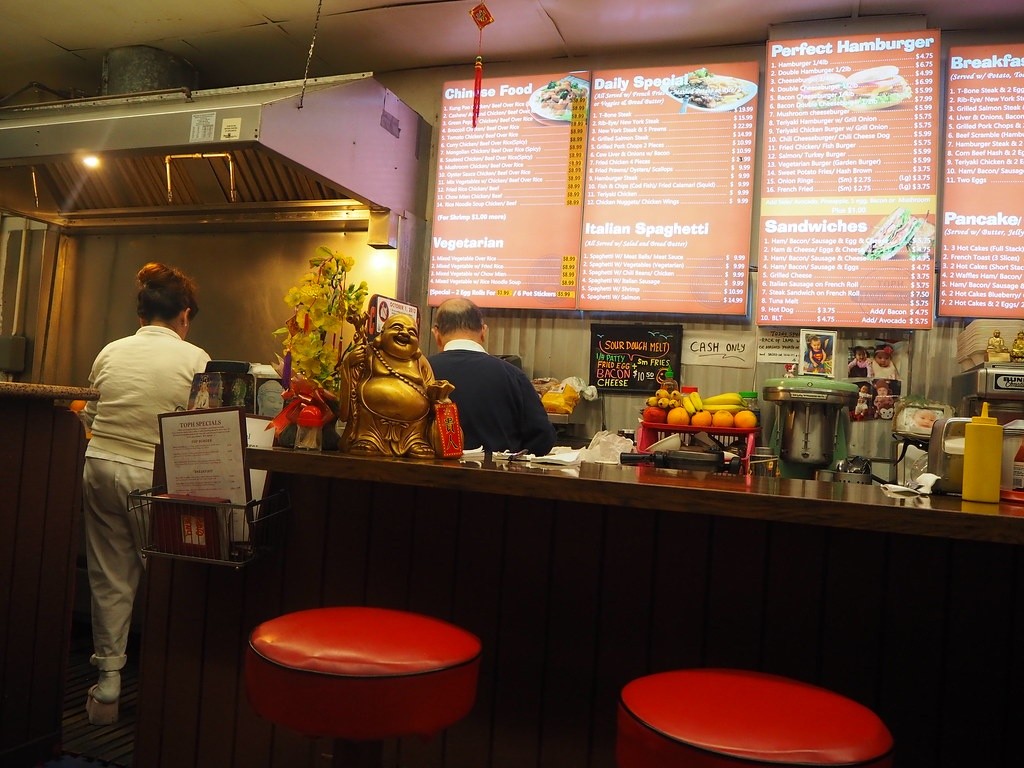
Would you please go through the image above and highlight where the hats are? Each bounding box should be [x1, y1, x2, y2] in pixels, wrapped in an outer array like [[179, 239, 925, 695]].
[[876, 379, 890, 392]]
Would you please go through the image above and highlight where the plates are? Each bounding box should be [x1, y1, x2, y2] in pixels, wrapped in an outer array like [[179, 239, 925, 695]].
[[528, 74, 590, 124], [660, 73, 758, 112]]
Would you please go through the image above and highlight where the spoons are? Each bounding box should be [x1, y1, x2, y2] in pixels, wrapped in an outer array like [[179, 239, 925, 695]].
[[679, 94, 692, 115]]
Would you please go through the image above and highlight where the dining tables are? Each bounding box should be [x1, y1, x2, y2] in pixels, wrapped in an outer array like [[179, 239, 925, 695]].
[[240, 430, 1023, 768]]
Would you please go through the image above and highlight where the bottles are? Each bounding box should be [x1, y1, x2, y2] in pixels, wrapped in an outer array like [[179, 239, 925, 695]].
[[661, 366, 679, 395], [293, 405, 322, 451], [962, 402, 1002, 503], [740, 391, 761, 428], [679, 386, 697, 395]]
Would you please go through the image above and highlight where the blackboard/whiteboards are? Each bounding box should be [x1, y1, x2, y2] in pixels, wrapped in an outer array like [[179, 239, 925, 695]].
[[587, 323, 683, 393]]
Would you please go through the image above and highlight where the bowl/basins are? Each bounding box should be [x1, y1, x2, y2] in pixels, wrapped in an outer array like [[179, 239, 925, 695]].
[[645, 434, 680, 456], [956, 317, 1024, 373]]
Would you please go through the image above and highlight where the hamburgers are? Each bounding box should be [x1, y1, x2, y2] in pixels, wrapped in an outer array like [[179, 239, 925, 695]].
[[861, 208, 924, 260], [799, 66, 913, 112]]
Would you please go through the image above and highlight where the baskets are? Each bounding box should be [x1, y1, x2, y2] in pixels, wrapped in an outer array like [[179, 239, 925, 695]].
[[124, 484, 270, 571]]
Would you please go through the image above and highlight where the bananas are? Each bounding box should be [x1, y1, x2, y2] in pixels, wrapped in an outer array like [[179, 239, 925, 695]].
[[645, 388, 748, 415]]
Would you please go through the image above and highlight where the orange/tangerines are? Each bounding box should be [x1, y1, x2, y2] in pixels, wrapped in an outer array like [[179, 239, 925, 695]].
[[666, 407, 758, 428]]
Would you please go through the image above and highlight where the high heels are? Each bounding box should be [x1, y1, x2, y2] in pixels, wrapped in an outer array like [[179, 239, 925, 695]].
[[82, 671, 125, 727]]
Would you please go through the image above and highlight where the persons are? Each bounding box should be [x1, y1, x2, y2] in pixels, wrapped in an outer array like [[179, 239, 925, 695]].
[[850, 382, 870, 417], [845, 345, 874, 381], [420, 295, 557, 468], [69, 260, 220, 735], [804, 336, 826, 378], [905, 410, 941, 435], [866, 343, 899, 382], [870, 380, 896, 417]]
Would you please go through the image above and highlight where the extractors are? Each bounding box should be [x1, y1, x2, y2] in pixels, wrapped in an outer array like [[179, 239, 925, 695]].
[[1, 46, 432, 249]]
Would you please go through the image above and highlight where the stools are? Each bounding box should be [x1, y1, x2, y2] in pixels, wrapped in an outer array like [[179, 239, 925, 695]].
[[613, 669, 901, 768], [243, 600, 483, 768]]
